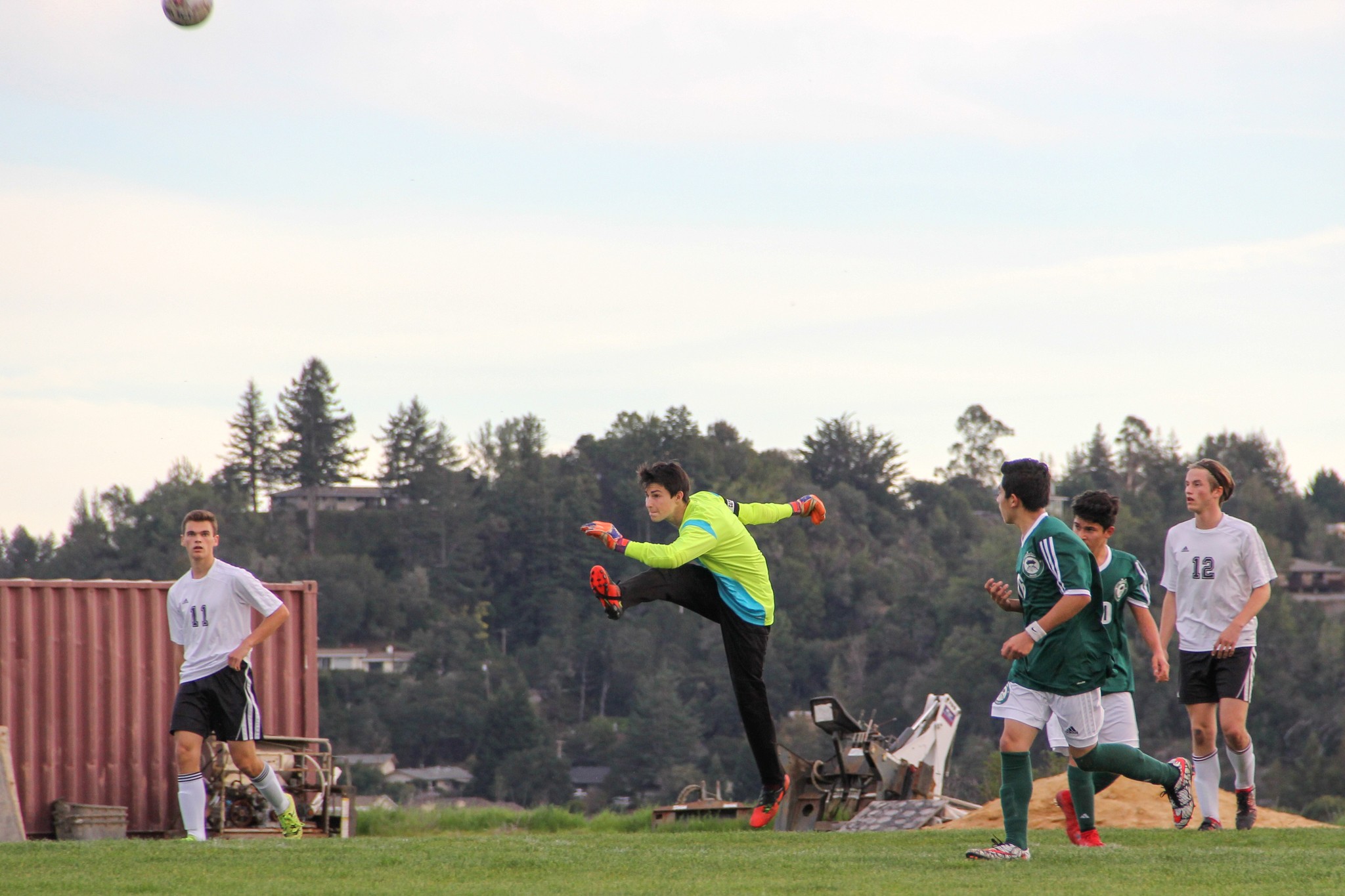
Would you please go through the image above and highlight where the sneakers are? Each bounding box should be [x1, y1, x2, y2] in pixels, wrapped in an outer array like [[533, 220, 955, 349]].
[[1198, 817, 1223, 831], [1054, 790, 1081, 846], [1159, 757, 1196, 829], [590, 565, 625, 619], [1234, 783, 1257, 829], [179, 834, 208, 843], [965, 833, 1030, 860], [276, 793, 305, 841], [750, 772, 789, 827], [1081, 828, 1106, 848]]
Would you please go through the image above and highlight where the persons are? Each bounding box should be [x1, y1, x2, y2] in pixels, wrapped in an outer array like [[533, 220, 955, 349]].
[[580, 459, 826, 828], [1158, 458, 1278, 831], [1054, 488, 1170, 846], [964, 459, 1195, 864], [166, 508, 304, 841]]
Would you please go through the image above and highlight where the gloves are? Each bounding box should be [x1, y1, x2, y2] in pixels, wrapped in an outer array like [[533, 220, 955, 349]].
[[792, 495, 827, 525], [580, 521, 630, 555]]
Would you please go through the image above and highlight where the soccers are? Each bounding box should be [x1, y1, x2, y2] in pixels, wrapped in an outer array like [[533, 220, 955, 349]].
[[160, 0, 214, 27]]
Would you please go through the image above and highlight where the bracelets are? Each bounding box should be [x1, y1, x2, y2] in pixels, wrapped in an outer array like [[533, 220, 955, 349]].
[[1024, 621, 1048, 642]]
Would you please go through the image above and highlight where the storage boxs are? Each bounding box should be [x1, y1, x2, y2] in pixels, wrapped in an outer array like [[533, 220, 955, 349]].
[[0, 578, 317, 841]]
